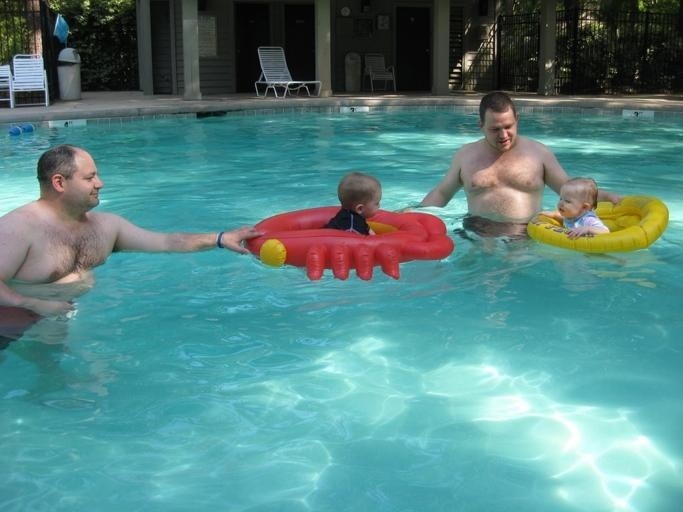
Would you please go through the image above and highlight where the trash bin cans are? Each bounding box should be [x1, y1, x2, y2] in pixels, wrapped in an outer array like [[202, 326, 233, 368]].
[[56, 46, 82, 101]]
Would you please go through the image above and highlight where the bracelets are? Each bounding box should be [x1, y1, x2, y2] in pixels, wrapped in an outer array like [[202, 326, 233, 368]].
[[216, 231, 224, 249]]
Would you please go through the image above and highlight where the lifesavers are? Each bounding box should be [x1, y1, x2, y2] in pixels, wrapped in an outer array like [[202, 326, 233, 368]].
[[527, 195, 668, 252], [247, 207, 452, 280]]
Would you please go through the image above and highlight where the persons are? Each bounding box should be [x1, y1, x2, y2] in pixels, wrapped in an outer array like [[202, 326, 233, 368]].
[[537, 175, 609, 240], [323, 169, 381, 236], [0, 142, 266, 352], [412, 90, 619, 254]]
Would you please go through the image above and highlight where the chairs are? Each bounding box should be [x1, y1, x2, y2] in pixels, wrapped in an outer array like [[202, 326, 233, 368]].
[[0, 53, 49, 109], [252, 46, 322, 99], [361, 53, 395, 93]]
